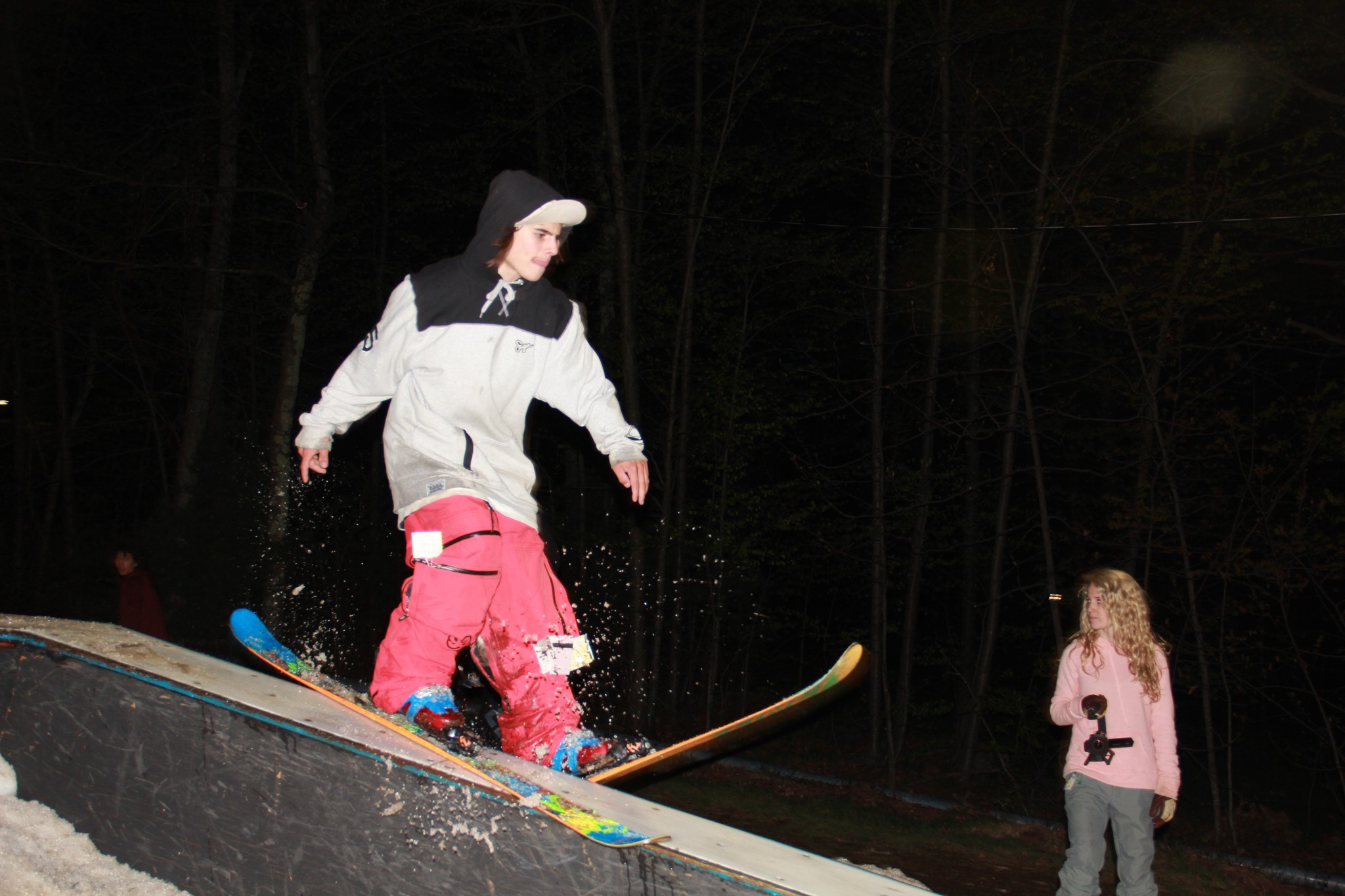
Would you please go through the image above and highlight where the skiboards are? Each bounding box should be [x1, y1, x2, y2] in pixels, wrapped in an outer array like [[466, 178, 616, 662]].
[[231, 607, 864, 848]]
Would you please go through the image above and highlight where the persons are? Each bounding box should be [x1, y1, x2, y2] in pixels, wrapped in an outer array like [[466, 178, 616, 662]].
[[1049, 570, 1181, 896], [295, 171, 657, 774], [110, 542, 166, 640]]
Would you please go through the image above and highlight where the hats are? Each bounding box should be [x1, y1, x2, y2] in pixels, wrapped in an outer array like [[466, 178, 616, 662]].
[[515, 200, 587, 230]]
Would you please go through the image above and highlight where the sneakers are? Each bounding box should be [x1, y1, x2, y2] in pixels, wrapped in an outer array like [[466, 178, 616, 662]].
[[401, 692, 482, 758], [557, 731, 650, 777]]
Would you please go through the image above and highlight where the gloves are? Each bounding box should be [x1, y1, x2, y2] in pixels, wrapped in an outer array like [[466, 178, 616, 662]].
[[1148, 794, 1177, 829], [1082, 694, 1108, 719]]
